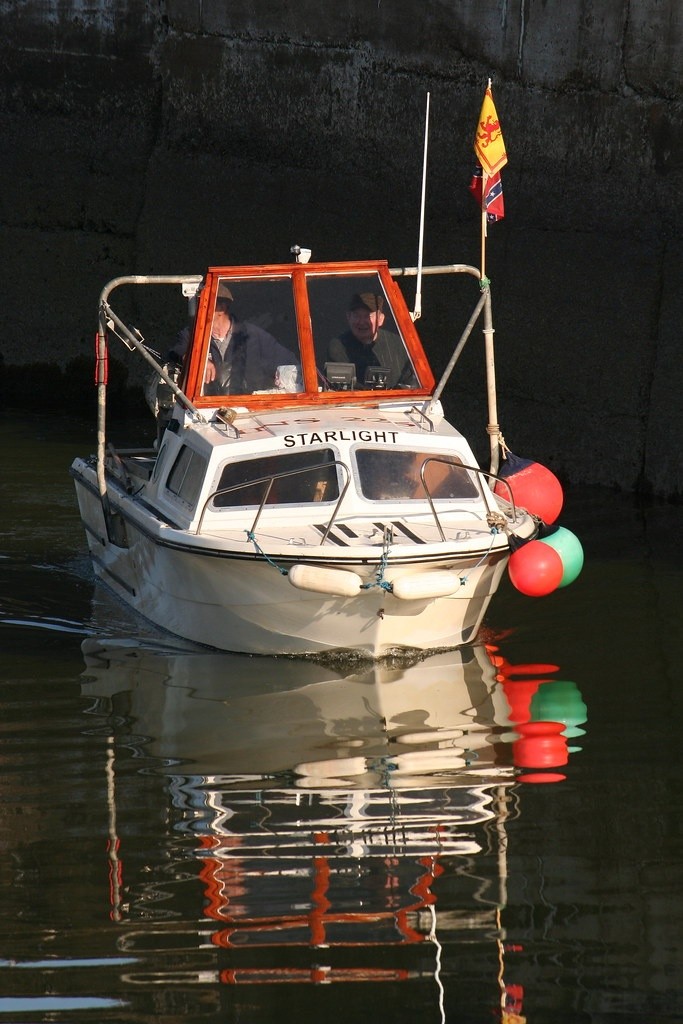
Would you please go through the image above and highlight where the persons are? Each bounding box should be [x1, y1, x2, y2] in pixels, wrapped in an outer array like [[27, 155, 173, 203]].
[[177, 284, 303, 396], [323, 292, 420, 391]]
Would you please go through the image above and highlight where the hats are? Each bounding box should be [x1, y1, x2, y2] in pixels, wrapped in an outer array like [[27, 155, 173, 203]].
[[198, 283, 234, 303], [348, 291, 384, 312]]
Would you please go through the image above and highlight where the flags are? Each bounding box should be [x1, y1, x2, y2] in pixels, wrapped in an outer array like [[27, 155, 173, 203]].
[[471, 87, 508, 222]]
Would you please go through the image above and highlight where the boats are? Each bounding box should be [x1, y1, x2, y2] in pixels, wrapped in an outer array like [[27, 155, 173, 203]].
[[73, 650, 551, 1023], [71, 94, 510, 660]]
